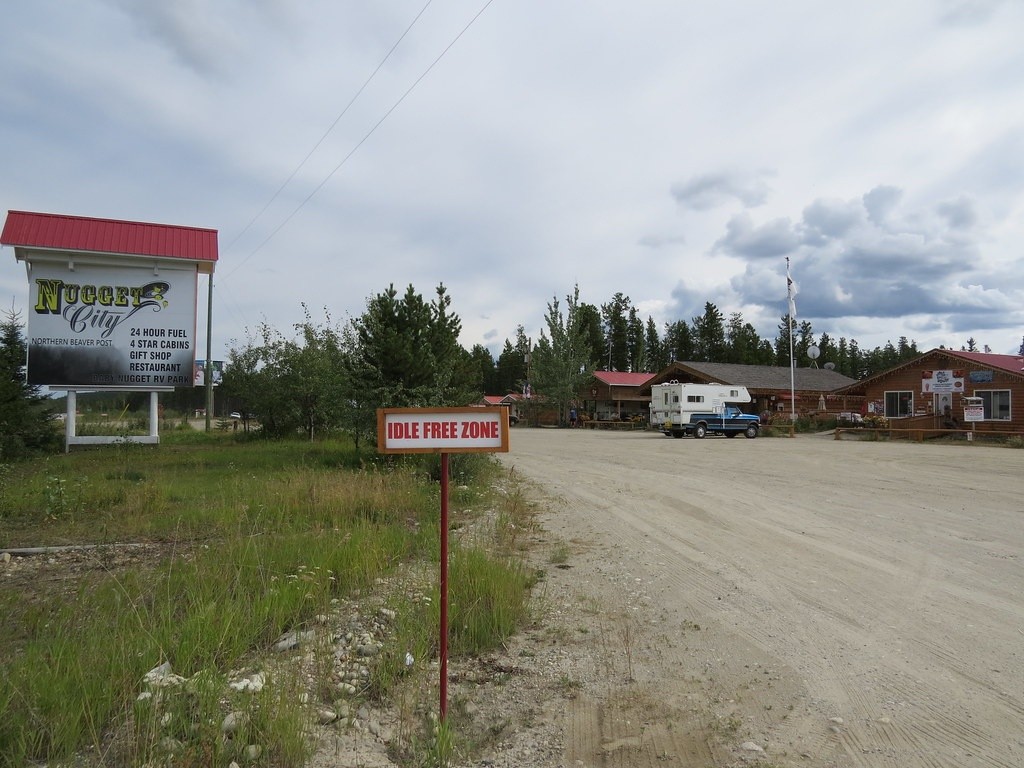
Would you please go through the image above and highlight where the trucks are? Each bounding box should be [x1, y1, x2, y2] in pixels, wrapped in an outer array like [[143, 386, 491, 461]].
[[649, 379, 760, 440]]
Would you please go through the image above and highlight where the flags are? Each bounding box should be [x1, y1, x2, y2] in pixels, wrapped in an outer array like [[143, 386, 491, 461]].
[[786, 268, 800, 318]]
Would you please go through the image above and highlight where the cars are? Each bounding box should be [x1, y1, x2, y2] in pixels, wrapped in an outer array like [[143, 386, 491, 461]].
[[508, 415, 520, 427], [230, 412, 243, 420]]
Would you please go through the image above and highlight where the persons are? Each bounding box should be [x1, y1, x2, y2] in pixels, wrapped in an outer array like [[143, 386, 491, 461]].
[[568, 405, 649, 427]]
